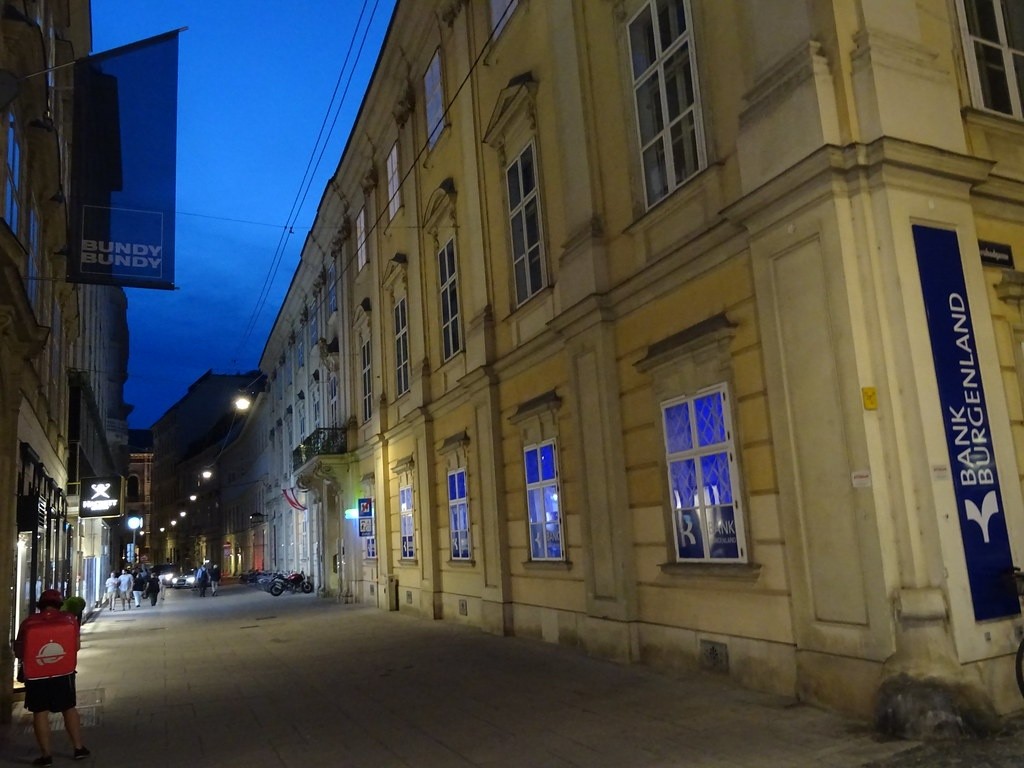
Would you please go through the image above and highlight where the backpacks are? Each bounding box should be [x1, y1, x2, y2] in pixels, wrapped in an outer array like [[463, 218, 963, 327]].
[[201, 568, 208, 582], [20, 611, 77, 681]]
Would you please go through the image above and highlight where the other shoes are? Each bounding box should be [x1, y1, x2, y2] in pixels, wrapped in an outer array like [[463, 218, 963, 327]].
[[34, 756, 52, 766], [212, 591, 216, 596], [74, 745, 89, 759]]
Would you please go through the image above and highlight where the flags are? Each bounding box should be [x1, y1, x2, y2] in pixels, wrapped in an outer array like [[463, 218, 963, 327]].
[[282, 487, 308, 511]]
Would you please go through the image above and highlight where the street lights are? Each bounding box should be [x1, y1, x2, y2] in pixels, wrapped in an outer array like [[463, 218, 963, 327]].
[[128, 516, 140, 561]]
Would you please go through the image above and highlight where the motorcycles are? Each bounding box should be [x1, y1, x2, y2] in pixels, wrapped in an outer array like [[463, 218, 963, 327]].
[[237, 567, 313, 596]]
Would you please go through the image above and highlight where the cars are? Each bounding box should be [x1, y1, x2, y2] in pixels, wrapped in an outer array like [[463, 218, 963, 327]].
[[153, 564, 210, 588]]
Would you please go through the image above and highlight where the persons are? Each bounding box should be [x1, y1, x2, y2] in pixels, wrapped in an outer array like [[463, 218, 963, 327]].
[[13, 589, 90, 767], [192, 561, 221, 597], [106, 566, 165, 611]]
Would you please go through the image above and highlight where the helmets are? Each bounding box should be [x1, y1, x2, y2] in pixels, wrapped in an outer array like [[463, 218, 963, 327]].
[[39, 590, 64, 606]]
